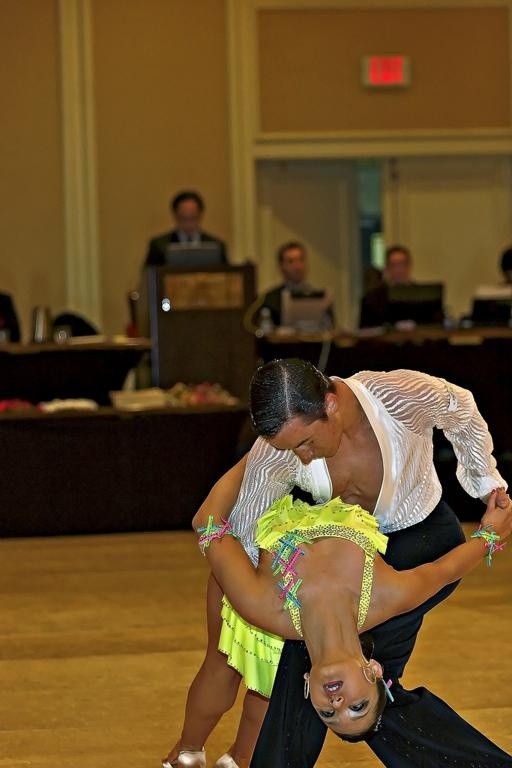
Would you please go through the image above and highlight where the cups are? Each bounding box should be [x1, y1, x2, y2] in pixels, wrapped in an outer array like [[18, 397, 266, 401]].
[[54, 327, 72, 345]]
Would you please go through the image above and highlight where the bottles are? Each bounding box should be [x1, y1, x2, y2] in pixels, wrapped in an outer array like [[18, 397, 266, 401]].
[[30, 304, 53, 344], [258, 308, 273, 335]]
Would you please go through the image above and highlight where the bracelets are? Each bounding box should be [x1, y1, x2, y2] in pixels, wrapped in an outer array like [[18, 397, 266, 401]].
[[470, 525, 509, 565]]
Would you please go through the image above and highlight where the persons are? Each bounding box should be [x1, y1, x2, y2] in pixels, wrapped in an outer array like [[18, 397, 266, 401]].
[[144, 191, 225, 267], [225, 359, 512, 768], [0, 290, 20, 343], [163, 448, 512, 768], [360, 246, 444, 328], [474, 247, 512, 300], [263, 241, 334, 330]]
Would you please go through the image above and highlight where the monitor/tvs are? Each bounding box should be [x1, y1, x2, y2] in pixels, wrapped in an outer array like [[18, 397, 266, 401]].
[[165, 243, 224, 271], [382, 281, 444, 326], [471, 296, 512, 327]]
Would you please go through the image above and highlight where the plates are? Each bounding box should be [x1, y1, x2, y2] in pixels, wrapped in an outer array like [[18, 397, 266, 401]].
[[107, 388, 166, 412]]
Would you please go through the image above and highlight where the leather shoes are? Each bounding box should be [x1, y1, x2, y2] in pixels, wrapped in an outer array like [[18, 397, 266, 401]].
[[161, 745, 207, 768], [213, 753, 240, 768]]
